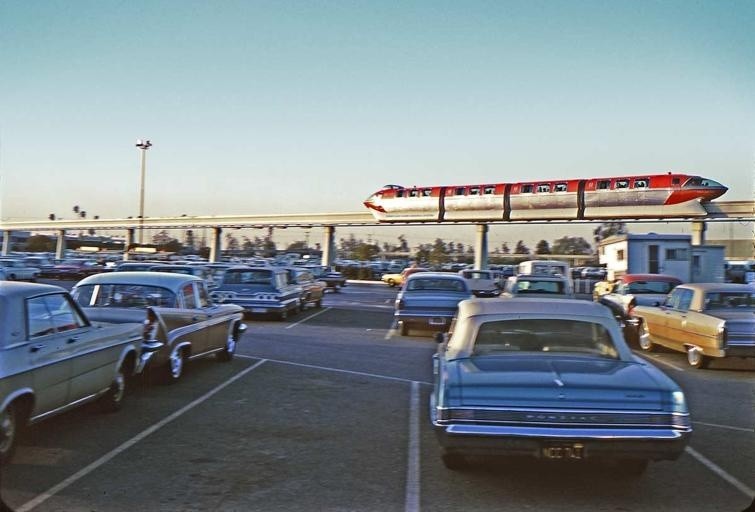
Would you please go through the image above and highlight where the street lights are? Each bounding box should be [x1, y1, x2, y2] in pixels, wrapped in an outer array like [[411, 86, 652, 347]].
[[135, 138, 152, 244]]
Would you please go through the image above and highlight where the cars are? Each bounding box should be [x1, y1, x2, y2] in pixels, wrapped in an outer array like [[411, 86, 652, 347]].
[[0, 280, 145, 468], [61, 272, 249, 387], [430, 295, 691, 471], [629, 282, 755, 369]]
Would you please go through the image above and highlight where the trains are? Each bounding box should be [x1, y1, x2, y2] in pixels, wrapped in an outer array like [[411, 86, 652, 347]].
[[362, 171, 729, 222]]
